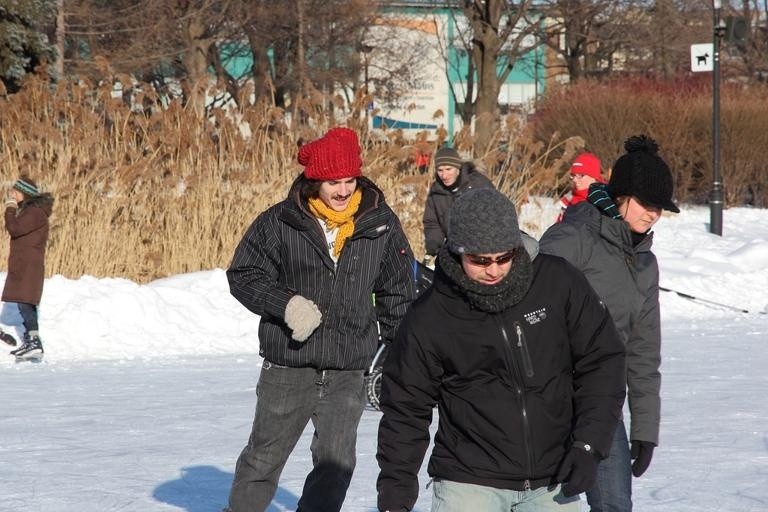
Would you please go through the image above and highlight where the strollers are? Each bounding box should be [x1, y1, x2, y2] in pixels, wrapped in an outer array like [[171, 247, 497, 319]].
[[355, 253, 437, 410]]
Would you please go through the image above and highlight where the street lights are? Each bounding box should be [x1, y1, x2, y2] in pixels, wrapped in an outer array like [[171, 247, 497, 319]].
[[689, 0, 730, 236]]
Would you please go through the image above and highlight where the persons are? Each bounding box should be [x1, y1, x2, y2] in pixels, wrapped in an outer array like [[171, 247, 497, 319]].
[[414, 142, 433, 175], [225, 127, 418, 511], [422, 147, 497, 268], [372, 186, 629, 512], [1, 174, 55, 361], [540, 134, 681, 512], [556, 153, 612, 224]]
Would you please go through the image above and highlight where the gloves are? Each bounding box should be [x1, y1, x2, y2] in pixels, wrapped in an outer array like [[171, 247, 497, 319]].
[[630, 440, 655, 477], [283, 293, 323, 342], [556, 447, 601, 498]]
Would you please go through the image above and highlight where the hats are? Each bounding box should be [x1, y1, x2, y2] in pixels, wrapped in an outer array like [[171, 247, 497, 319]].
[[449, 188, 523, 255], [607, 149, 681, 213], [297, 127, 363, 183], [433, 147, 462, 171], [570, 153, 606, 184], [10, 176, 39, 199]]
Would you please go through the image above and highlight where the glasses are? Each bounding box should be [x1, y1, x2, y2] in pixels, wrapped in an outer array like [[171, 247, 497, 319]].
[[466, 252, 515, 266]]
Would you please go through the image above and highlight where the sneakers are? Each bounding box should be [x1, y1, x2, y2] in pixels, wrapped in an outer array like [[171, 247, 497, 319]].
[[10, 335, 44, 359]]
